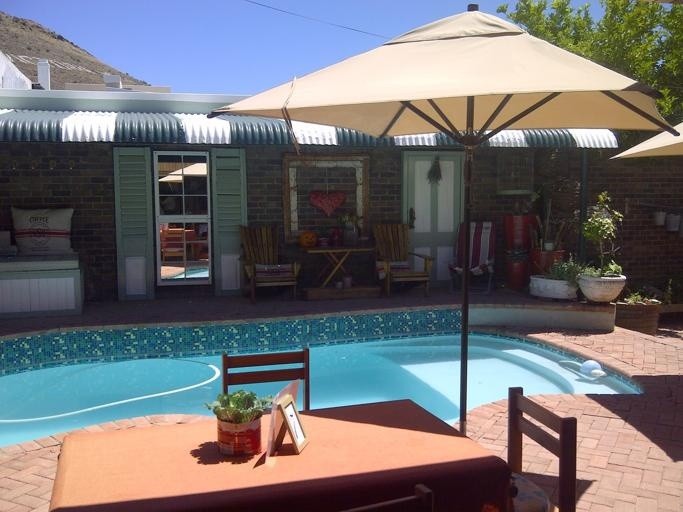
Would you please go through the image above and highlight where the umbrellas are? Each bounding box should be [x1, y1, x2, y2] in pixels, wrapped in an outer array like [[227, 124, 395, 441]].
[[206, 6, 680, 439], [608, 122, 682, 162]]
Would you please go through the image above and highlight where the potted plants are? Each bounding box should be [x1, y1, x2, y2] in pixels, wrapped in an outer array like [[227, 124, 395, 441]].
[[501, 180, 680, 336], [203, 389, 272, 457]]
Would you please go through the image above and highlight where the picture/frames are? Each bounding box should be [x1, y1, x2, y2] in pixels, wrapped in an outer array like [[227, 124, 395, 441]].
[[274, 396, 311, 456]]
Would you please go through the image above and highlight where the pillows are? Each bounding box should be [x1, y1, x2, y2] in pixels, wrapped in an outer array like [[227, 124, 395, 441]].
[[9, 205, 75, 253]]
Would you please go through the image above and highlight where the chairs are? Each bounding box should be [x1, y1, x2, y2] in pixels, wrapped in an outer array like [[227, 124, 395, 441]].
[[507, 384, 579, 512], [222, 348, 311, 413], [235, 223, 298, 304], [161, 228, 187, 267], [446, 218, 497, 298], [372, 221, 434, 301]]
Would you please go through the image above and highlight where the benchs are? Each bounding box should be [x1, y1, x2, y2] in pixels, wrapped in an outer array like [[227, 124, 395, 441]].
[[1, 252, 87, 321]]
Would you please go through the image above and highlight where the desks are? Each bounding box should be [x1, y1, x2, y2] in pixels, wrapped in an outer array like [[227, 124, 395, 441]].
[[303, 243, 376, 289], [48, 397, 509, 511]]
